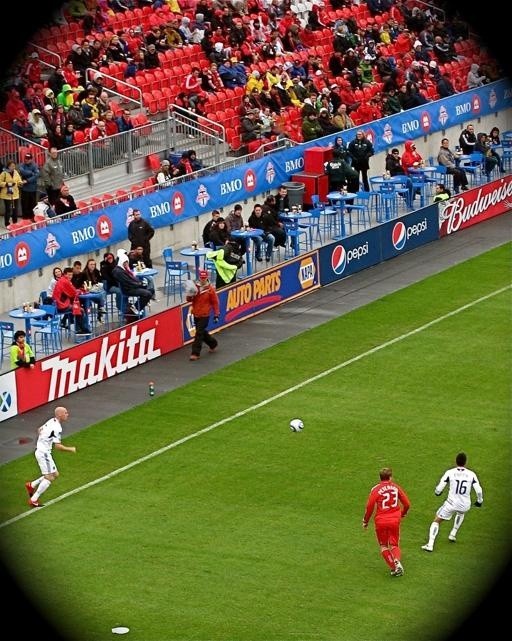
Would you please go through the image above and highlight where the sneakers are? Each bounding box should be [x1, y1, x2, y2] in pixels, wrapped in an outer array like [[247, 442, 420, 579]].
[[209, 348, 216, 352], [28, 499, 45, 507], [25, 482, 34, 498], [449, 535, 456, 540], [57, 305, 144, 336], [189, 355, 200, 360], [422, 545, 433, 551], [394, 559, 404, 576], [391, 570, 398, 577]]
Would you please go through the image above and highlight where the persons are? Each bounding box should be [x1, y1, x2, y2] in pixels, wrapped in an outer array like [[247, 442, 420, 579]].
[[1, 0, 512, 371], [362, 467, 410, 577], [24, 406, 76, 508], [421, 452, 484, 552]]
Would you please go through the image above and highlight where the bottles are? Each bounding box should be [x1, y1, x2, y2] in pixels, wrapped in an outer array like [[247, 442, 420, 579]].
[[132, 260, 145, 272], [284, 203, 302, 216], [489, 137, 493, 145], [148, 382, 155, 396], [83, 280, 93, 293], [191, 240, 198, 252], [382, 169, 391, 180], [22, 302, 32, 315], [340, 185, 347, 196], [421, 160, 425, 168]]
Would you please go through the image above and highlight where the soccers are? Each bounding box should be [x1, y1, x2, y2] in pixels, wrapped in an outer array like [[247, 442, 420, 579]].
[[290, 418, 303, 430]]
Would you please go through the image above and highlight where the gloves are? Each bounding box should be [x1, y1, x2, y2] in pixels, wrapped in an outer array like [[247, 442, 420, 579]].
[[214, 316, 219, 323], [474, 502, 481, 507]]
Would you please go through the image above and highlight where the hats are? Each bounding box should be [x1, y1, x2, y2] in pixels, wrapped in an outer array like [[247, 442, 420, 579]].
[[39, 193, 48, 201], [162, 160, 170, 166], [199, 270, 208, 277], [182, 151, 189, 158], [24, 153, 32, 159]]
